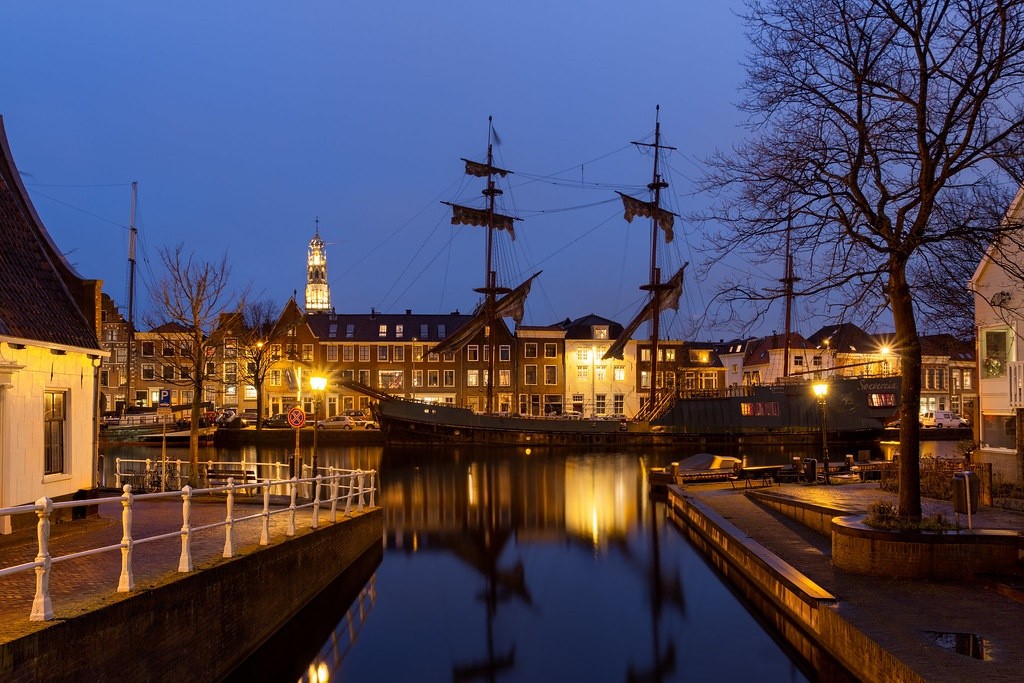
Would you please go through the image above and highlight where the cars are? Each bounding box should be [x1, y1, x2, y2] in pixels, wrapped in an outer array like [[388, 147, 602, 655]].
[[262, 413, 291, 429], [334, 409, 367, 423], [364, 420, 382, 430]]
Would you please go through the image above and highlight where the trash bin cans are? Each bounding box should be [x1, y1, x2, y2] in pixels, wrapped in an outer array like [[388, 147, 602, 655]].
[[803, 458, 818, 484], [954, 472, 980, 513]]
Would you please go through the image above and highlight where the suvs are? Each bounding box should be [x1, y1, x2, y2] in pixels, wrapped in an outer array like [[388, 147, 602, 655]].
[[312, 415, 356, 431]]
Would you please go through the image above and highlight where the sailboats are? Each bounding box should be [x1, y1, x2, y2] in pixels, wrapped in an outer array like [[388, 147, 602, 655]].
[[336, 104, 726, 446]]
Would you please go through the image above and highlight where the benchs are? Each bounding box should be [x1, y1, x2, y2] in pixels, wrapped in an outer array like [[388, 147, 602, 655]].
[[730, 465, 784, 488], [197, 466, 256, 497]]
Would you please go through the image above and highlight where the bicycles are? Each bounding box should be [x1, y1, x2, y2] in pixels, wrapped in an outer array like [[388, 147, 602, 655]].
[[141, 454, 183, 494]]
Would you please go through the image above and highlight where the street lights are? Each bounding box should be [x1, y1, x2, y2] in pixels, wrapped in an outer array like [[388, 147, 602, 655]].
[[813, 381, 835, 485]]
